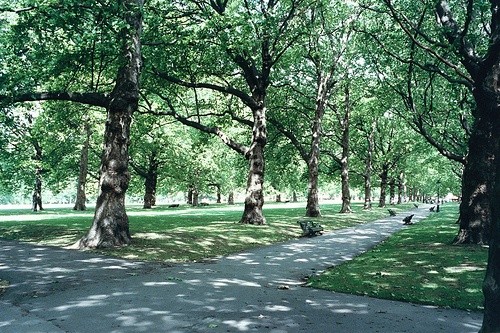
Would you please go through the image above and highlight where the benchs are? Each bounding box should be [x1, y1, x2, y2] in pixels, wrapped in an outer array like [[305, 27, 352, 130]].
[[297, 220, 324, 238]]
